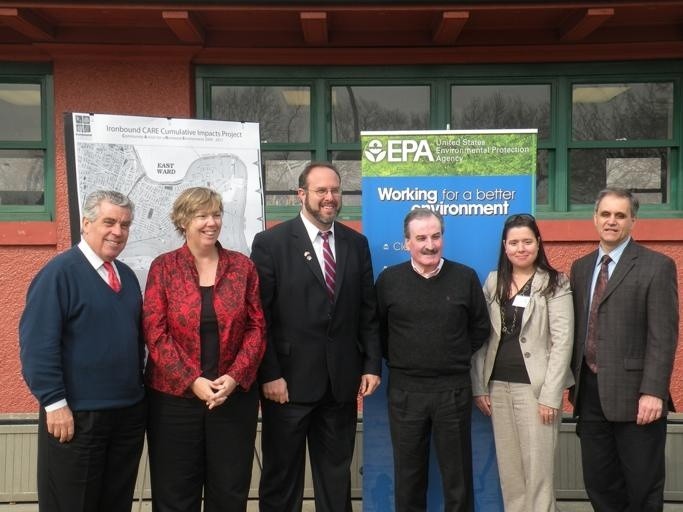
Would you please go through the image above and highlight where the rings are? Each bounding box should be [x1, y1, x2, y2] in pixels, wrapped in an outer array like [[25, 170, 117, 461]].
[[548, 411, 553, 415]]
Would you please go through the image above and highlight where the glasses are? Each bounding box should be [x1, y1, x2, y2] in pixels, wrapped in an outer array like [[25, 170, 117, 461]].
[[303, 188, 338, 197]]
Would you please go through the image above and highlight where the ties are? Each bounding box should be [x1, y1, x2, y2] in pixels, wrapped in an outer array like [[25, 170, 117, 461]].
[[104, 261, 121, 294], [319, 232, 336, 304], [585, 255, 613, 373]]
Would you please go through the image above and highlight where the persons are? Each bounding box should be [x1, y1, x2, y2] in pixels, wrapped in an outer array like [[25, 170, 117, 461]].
[[141, 186, 268, 512], [569, 186, 678, 511], [248, 162, 380, 511], [372, 208, 496, 511], [17, 187, 145, 512], [470, 209, 578, 511]]
